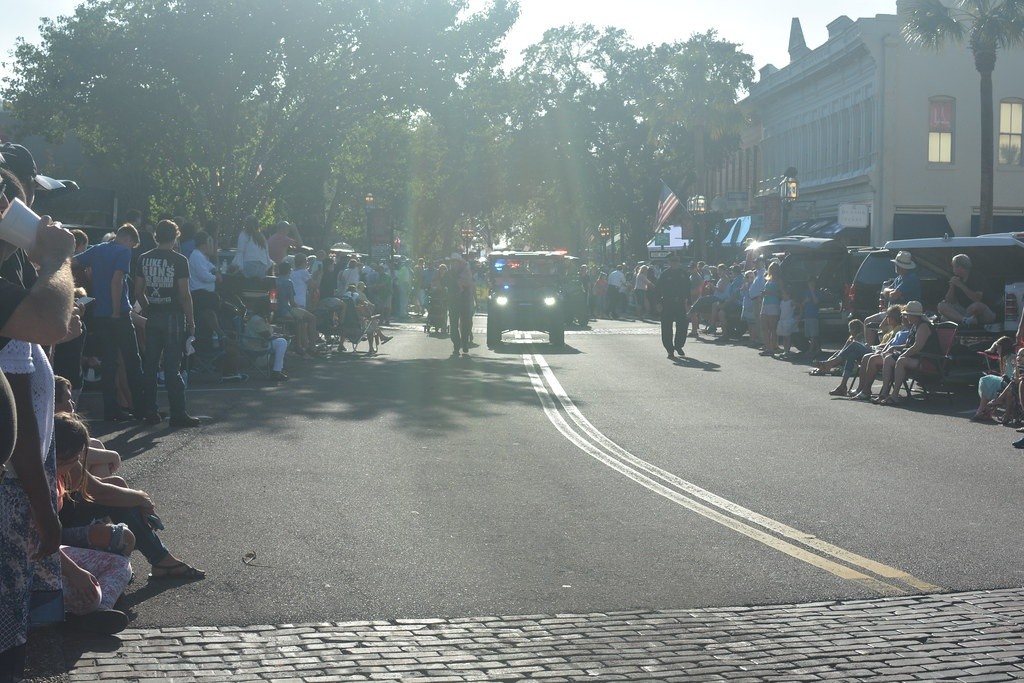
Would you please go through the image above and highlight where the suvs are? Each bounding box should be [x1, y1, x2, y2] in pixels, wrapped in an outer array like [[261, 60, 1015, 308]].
[[848, 232, 1024, 381], [486, 250, 569, 348], [741, 236, 887, 351]]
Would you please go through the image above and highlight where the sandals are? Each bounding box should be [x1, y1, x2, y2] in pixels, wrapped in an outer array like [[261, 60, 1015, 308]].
[[148, 562, 206, 579]]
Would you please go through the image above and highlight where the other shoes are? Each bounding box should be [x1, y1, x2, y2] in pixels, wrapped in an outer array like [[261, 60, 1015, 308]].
[[272, 372, 288, 380], [219, 373, 248, 383], [147, 412, 160, 424], [585, 312, 816, 360], [66, 611, 128, 634], [871, 395, 900, 405], [971, 399, 1023, 448], [668, 354, 674, 360], [170, 418, 198, 426], [675, 346, 684, 355], [814, 362, 830, 373], [291, 314, 474, 358], [104, 411, 134, 421], [688, 333, 698, 336], [849, 389, 872, 400]]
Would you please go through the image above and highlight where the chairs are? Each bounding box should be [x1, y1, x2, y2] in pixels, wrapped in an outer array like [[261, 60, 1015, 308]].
[[185, 284, 381, 385], [977, 335, 1024, 428], [846, 321, 959, 412]]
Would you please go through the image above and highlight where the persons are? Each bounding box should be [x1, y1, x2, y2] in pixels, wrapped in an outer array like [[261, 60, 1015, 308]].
[[1, 138, 481, 682], [564, 242, 1023, 444]]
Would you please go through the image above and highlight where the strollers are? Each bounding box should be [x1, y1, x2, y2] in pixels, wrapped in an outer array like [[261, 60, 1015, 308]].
[[977, 308, 1024, 424], [424, 288, 450, 333]]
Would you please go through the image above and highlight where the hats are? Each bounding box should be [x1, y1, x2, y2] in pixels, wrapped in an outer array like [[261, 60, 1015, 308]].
[[891, 251, 915, 269], [0, 143, 37, 176], [901, 302, 925, 315]]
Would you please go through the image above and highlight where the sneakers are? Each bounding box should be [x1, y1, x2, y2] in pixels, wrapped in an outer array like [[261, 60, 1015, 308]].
[[830, 385, 847, 394], [962, 316, 979, 326]]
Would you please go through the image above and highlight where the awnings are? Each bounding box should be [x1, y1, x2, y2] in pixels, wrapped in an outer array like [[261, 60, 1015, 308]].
[[766, 216, 850, 240], [645, 214, 755, 251], [653, 183, 679, 234]]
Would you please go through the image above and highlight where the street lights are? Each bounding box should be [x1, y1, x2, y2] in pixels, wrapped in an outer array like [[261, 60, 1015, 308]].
[[364, 191, 375, 240], [686, 193, 707, 256], [779, 167, 800, 231]]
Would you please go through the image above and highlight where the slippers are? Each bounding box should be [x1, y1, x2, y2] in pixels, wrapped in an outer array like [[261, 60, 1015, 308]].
[[810, 369, 825, 376]]
